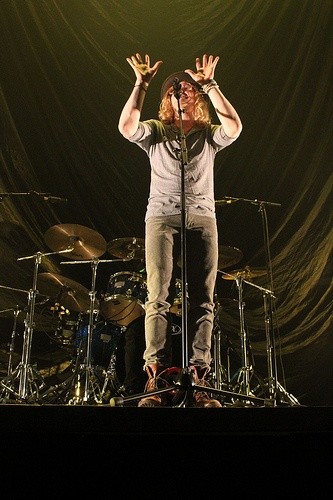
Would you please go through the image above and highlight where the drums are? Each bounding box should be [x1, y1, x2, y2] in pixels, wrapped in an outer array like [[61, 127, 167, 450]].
[[100, 270, 147, 329], [63, 363, 113, 405], [167, 277, 189, 336]]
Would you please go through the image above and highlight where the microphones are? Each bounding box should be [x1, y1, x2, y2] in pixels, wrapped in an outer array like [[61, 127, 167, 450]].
[[42, 196, 68, 204], [214, 199, 235, 206], [173, 78, 182, 91]]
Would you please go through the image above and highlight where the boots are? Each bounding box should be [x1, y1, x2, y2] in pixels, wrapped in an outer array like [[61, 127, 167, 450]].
[[138, 363, 168, 407], [187, 365, 222, 408]]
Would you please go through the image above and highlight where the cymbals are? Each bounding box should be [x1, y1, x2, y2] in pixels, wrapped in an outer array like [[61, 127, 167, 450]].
[[44, 224, 107, 260], [106, 237, 145, 259], [33, 272, 91, 313], [218, 245, 244, 270], [221, 267, 272, 280]]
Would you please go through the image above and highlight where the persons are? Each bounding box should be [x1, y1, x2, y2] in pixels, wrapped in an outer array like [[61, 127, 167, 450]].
[[124, 312, 171, 407], [119, 53, 243, 407]]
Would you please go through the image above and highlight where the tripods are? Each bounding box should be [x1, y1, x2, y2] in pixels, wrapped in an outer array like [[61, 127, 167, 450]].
[[0, 240, 136, 403], [110, 88, 300, 406]]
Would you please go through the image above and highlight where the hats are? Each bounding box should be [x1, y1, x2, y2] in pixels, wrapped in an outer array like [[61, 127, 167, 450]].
[[160, 70, 201, 99]]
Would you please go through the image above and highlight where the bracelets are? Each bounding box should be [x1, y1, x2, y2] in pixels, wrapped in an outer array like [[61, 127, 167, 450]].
[[203, 80, 219, 93], [134, 85, 146, 92]]
